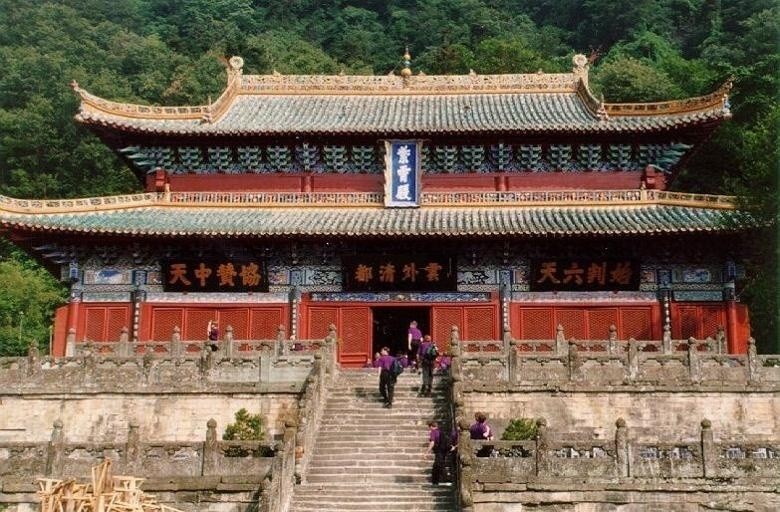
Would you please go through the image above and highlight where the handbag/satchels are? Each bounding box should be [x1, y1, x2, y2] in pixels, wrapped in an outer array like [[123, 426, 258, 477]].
[[391, 360, 403, 376], [426, 345, 437, 361], [434, 429, 449, 453]]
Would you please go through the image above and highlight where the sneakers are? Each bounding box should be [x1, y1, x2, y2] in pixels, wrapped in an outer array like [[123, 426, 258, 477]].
[[380, 398, 392, 407], [417, 393, 432, 397]]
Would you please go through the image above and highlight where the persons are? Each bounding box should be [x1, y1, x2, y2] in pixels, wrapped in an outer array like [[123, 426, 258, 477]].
[[449, 427, 460, 452], [469, 412, 494, 457], [408, 321, 423, 365], [364, 336, 450, 373], [377, 347, 397, 408], [415, 335, 437, 396], [476, 415, 489, 440], [206, 320, 219, 351], [424, 422, 453, 485]]
